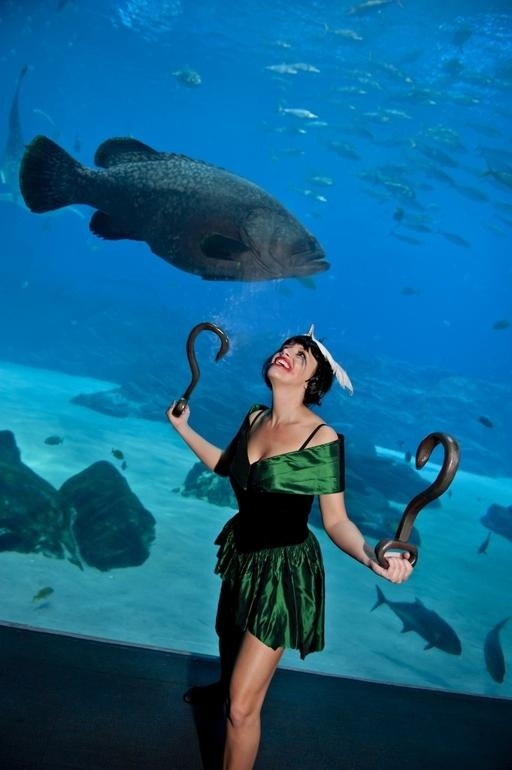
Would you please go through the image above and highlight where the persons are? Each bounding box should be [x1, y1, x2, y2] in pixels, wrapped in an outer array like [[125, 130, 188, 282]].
[[165, 330, 416, 770]]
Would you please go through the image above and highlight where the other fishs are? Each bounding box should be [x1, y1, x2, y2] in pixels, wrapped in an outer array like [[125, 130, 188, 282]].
[[52, 2, 511, 249], [18, 134, 330, 287], [6, 63, 33, 161]]
